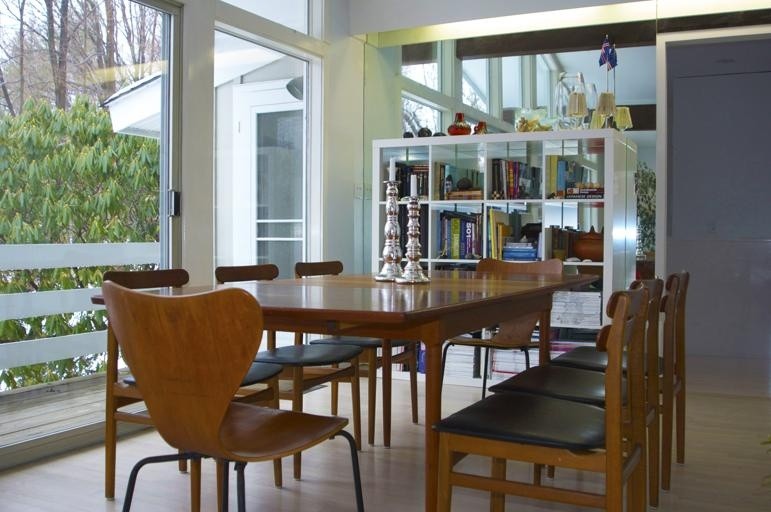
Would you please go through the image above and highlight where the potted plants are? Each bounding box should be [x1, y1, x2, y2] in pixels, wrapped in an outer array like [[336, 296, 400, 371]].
[[633, 159, 655, 283]]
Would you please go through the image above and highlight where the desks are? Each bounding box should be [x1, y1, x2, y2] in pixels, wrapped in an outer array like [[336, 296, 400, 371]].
[[89, 268, 610, 511]]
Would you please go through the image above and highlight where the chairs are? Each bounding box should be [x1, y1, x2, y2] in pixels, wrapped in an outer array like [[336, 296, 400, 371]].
[[487, 278, 665, 510], [440, 258, 561, 397], [432, 281, 652, 511], [97, 274, 367, 512], [293, 260, 419, 447], [545, 271, 690, 490], [212, 263, 363, 481], [101, 269, 285, 511]]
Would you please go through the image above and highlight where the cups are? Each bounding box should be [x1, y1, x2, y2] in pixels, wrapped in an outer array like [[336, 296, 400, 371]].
[[475, 122, 487, 134], [554, 249, 567, 261]]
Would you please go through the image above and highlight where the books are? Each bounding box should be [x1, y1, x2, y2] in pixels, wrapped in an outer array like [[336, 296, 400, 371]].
[[487, 205, 543, 262], [491, 159, 542, 199], [550, 155, 604, 199], [388, 327, 540, 383], [439, 211, 483, 259], [396, 162, 429, 258], [447, 190, 484, 200]]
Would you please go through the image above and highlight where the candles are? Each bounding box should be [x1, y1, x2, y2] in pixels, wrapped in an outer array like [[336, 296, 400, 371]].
[[408, 171, 419, 199], [388, 157, 398, 181]]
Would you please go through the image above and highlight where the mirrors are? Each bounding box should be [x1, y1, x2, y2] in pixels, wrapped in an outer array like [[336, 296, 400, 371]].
[[364, 11, 658, 281]]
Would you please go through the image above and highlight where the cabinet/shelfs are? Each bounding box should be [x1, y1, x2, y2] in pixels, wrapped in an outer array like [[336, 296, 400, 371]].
[[369, 127, 630, 350]]
